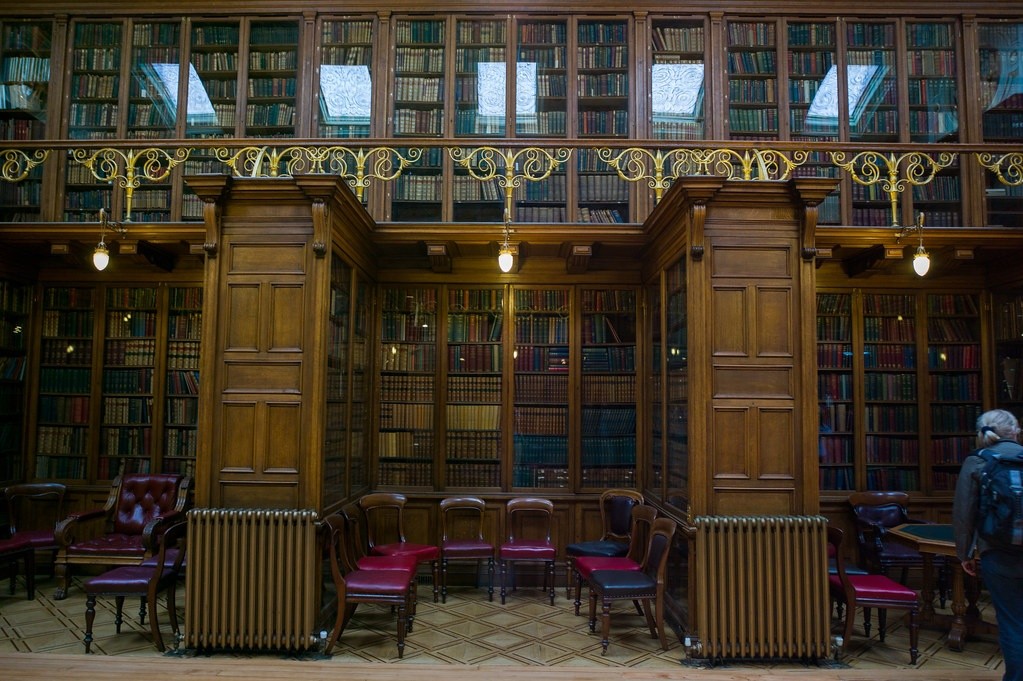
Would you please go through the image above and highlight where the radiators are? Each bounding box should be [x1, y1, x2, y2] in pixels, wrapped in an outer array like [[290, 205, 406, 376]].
[[687, 514, 845, 666], [184, 508, 325, 653]]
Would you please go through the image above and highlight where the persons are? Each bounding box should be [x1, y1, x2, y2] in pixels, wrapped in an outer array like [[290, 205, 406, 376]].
[[952, 409, 1022, 680]]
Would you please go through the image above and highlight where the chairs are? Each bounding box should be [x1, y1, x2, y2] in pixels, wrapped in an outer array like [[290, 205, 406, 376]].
[[342, 503, 418, 634], [361, 493, 439, 609], [847, 491, 947, 616], [588, 517, 677, 656], [322, 513, 413, 659], [573, 505, 658, 633], [499, 497, 557, 606], [0, 482, 68, 601], [439, 497, 496, 604], [82, 506, 191, 654], [52, 474, 190, 602], [825, 523, 921, 667], [564, 489, 644, 615]]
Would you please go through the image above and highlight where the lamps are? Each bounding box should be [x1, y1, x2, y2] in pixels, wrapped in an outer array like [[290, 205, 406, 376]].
[[896, 211, 931, 275], [93, 207, 126, 271], [495, 207, 513, 273]]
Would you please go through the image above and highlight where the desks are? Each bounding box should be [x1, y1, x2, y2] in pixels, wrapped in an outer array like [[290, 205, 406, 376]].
[[887, 522, 1005, 652]]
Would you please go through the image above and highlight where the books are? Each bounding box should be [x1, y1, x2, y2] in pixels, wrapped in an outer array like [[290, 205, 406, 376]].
[[0, 19, 1023, 492]]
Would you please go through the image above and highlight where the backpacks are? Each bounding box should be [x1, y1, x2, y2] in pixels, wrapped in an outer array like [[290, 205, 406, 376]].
[[975, 448, 1023, 553]]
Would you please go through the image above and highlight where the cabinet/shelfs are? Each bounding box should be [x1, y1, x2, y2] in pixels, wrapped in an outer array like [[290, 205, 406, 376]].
[[0, 0, 988, 229], [1, 247, 1023, 500]]
